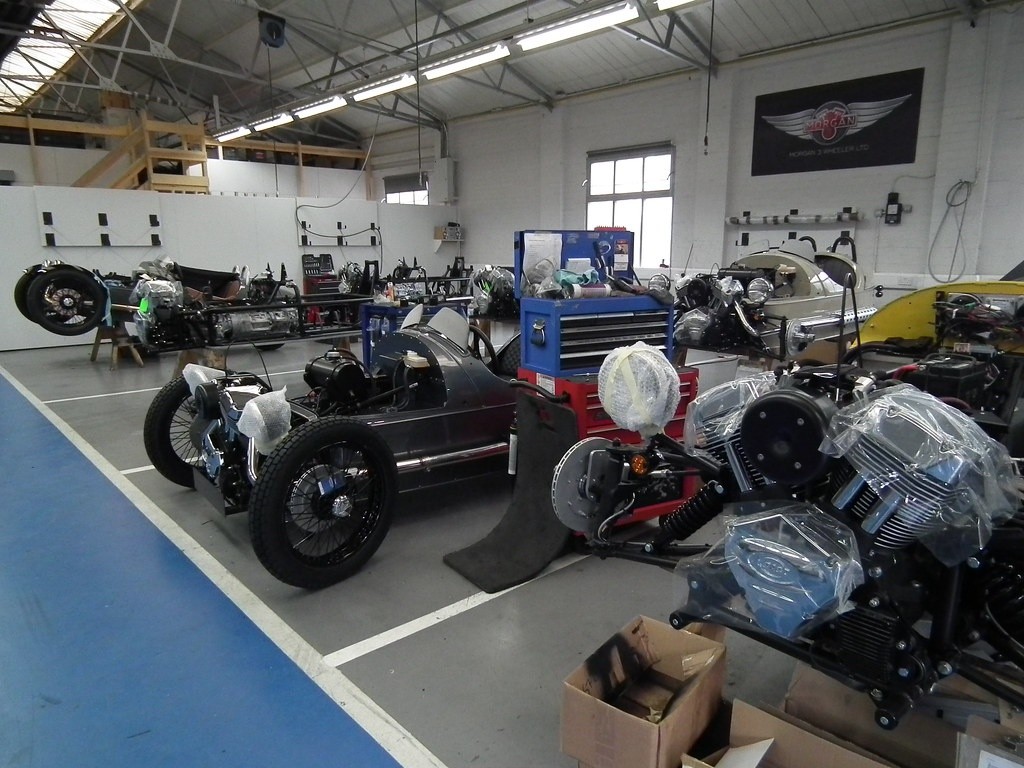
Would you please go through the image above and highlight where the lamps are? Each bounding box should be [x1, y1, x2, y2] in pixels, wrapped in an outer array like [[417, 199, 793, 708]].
[[511, 3, 639, 50], [421, 44, 513, 83], [292, 96, 349, 121], [348, 75, 419, 104], [217, 126, 252, 142], [252, 113, 294, 132]]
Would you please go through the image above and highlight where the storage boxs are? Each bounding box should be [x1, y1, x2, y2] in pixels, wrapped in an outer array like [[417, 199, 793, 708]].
[[678, 660, 1024, 768], [559, 613, 727, 768]]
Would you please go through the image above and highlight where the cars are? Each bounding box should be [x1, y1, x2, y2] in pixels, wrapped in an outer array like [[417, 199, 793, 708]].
[[13, 257, 301, 351], [144, 303, 740, 592]]
